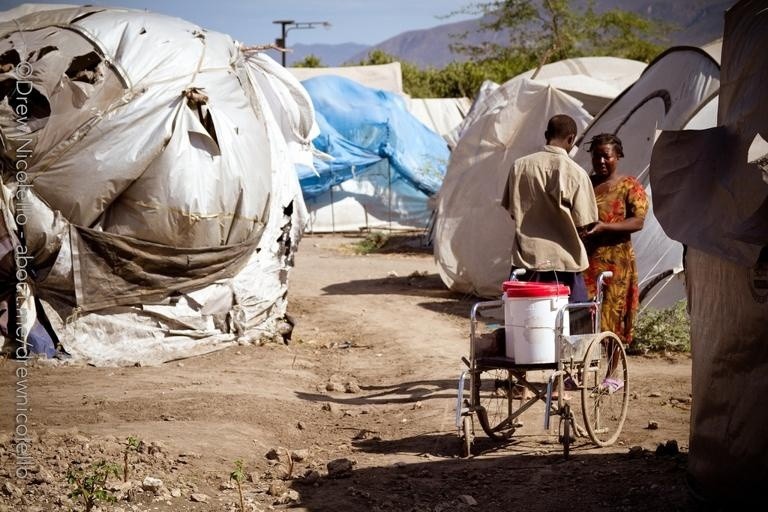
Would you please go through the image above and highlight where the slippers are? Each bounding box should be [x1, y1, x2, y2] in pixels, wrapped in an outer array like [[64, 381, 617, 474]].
[[552, 371, 624, 400]]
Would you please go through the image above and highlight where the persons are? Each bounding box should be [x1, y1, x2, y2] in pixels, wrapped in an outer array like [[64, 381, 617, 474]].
[[498, 112, 598, 307], [573, 133, 648, 395]]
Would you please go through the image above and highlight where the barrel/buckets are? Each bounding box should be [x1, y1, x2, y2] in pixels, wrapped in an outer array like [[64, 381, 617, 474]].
[[503, 261, 570, 365]]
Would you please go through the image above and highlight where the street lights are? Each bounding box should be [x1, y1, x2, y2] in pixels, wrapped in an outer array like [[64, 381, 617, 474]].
[[272, 19, 332, 67]]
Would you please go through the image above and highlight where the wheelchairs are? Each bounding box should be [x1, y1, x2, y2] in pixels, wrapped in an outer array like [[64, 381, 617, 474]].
[[454, 268, 629, 454]]
